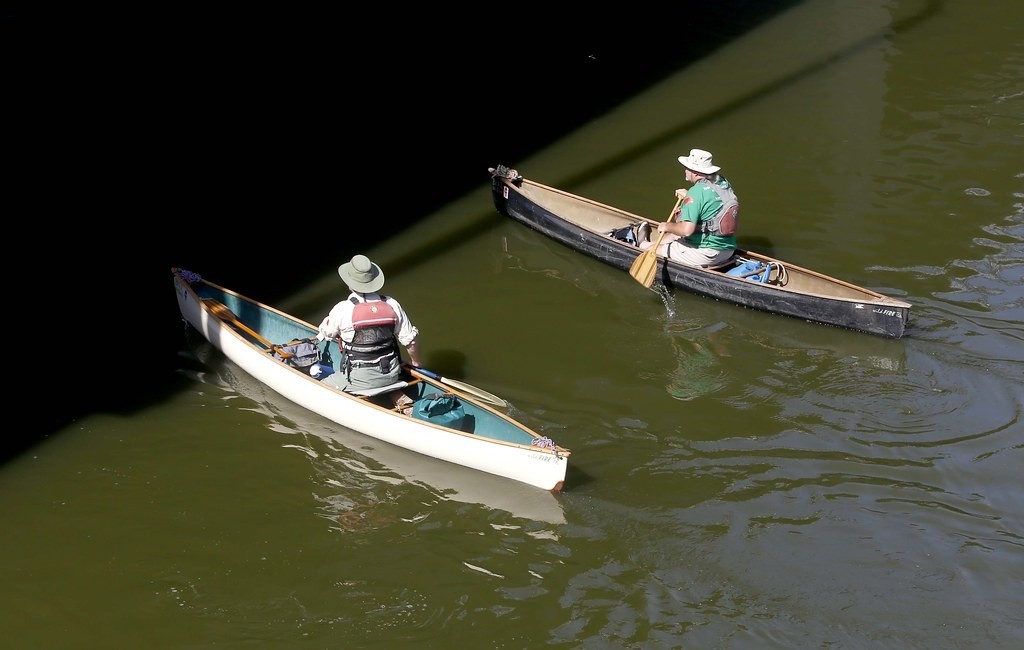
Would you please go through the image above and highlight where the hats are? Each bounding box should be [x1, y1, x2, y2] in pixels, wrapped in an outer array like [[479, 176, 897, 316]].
[[678, 149, 721, 174], [338, 255, 385, 293]]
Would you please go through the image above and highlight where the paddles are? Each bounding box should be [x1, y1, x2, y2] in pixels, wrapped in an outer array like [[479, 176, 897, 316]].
[[199, 296, 293, 359], [402, 361, 507, 407], [629, 194, 683, 290]]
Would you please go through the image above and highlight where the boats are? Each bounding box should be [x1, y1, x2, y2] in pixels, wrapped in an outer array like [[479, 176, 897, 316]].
[[487, 163, 912, 338], [163, 264, 571, 499]]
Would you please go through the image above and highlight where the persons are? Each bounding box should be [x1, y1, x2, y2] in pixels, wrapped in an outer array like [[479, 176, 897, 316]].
[[314, 255, 425, 393], [646, 148, 739, 267]]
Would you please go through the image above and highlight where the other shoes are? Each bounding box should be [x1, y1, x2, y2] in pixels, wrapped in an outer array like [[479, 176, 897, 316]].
[[637, 221, 649, 247]]
